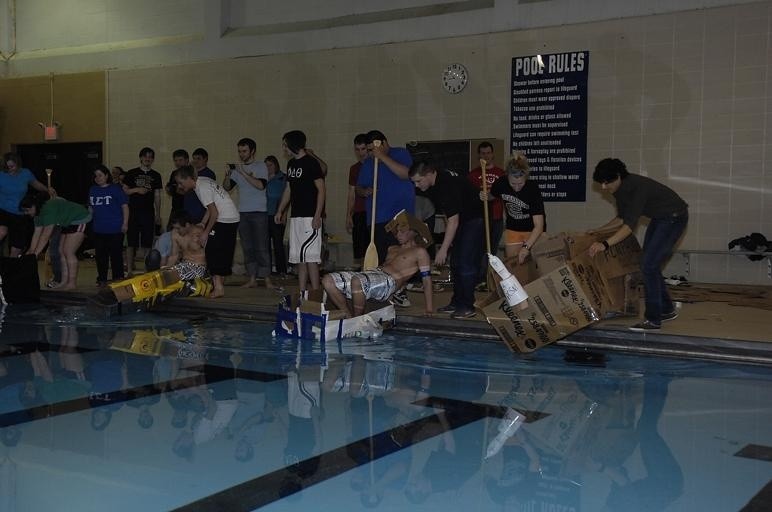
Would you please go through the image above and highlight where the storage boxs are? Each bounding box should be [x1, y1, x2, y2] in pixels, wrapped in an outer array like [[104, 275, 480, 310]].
[[473, 215, 650, 355], [270, 287, 396, 342]]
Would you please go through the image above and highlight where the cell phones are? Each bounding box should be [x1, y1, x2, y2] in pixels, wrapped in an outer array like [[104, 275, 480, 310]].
[[227, 163, 236, 170]]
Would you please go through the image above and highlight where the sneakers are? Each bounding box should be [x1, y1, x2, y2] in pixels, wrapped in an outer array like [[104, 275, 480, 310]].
[[438, 304, 455, 313], [629, 321, 661, 332], [393, 290, 410, 307], [660, 309, 679, 322], [451, 309, 474, 318]]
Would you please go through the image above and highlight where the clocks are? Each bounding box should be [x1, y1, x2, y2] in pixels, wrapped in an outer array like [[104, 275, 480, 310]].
[[442, 63, 469, 94]]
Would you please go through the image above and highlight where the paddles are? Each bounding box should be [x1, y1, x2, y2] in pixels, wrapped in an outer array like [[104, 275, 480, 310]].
[[44, 168, 55, 285], [364, 140, 381, 269], [480, 159, 496, 292]]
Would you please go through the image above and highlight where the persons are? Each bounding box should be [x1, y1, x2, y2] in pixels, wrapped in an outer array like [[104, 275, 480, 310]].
[[409, 158, 485, 320], [23, 134, 330, 298], [346, 133, 371, 271], [1, 322, 685, 512], [588, 158, 689, 332], [0, 152, 56, 257], [479, 158, 546, 266], [355, 131, 416, 308], [321, 213, 433, 318], [467, 142, 506, 292]]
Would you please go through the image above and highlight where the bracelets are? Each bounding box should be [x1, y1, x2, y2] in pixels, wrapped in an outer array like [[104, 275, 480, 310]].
[[522, 244, 532, 251], [602, 240, 609, 253]]
